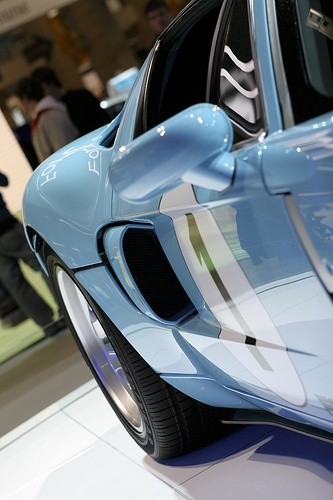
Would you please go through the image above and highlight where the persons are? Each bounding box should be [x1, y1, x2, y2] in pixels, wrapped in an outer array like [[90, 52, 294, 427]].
[[27, 66, 113, 141], [134, 0, 174, 71], [0, 170, 65, 334], [12, 75, 78, 165]]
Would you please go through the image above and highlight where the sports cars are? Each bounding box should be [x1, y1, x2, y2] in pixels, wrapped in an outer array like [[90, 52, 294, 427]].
[[21, 0, 333, 462]]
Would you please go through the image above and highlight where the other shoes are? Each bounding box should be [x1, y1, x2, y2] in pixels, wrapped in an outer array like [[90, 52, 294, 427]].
[[42, 320, 66, 336]]
